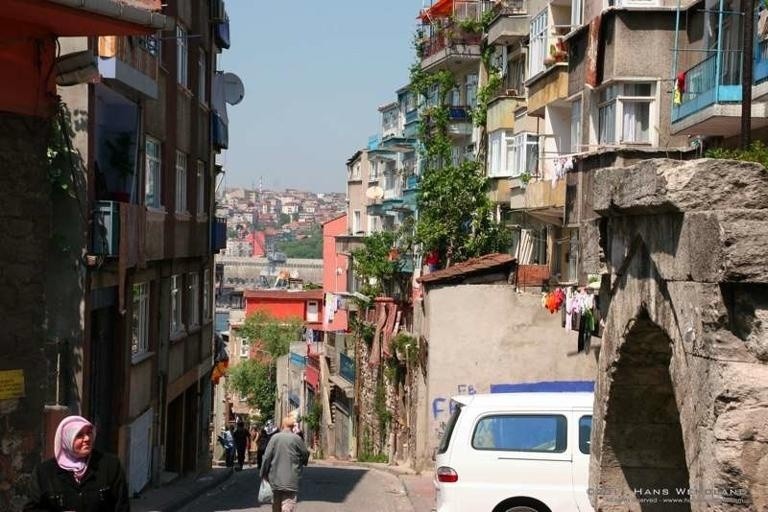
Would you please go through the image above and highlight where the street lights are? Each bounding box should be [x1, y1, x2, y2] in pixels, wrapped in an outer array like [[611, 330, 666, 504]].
[[323, 285, 372, 458]]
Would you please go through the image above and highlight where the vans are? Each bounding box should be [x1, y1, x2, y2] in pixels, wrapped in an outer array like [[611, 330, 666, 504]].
[[427, 387, 595, 512]]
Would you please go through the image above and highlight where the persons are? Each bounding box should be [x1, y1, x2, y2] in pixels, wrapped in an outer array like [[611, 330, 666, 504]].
[[253, 420, 266, 468], [222, 423, 236, 467], [23, 414, 131, 511], [246, 425, 258, 468], [256, 419, 282, 478], [291, 421, 306, 443], [258, 416, 311, 511], [232, 421, 252, 469]]
